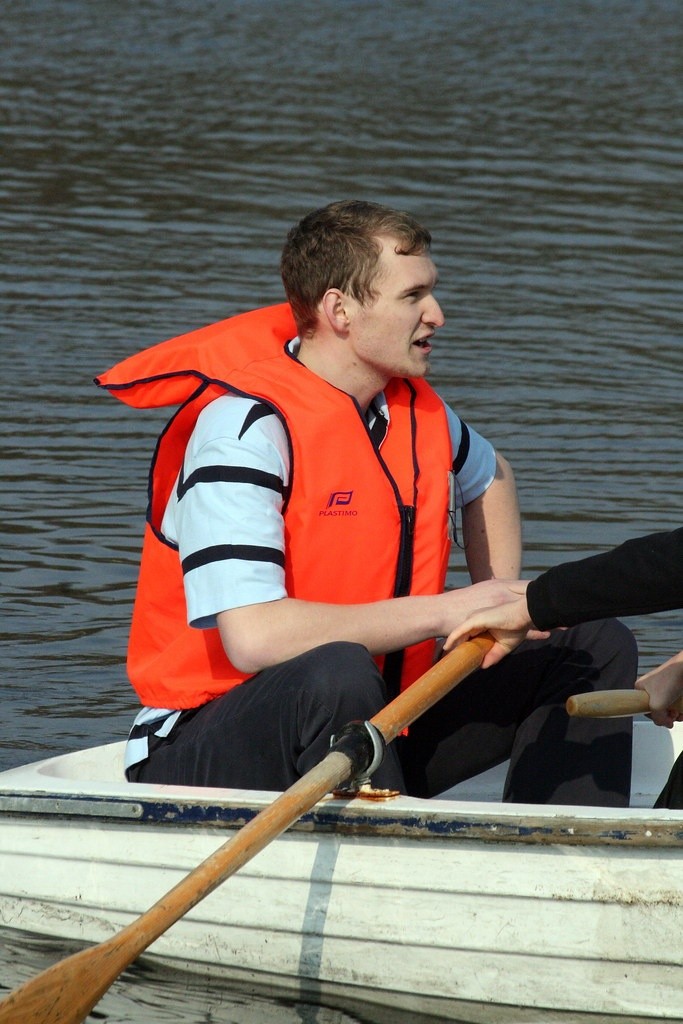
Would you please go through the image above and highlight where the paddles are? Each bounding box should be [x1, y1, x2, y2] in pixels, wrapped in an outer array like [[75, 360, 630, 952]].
[[565, 689, 683, 720], [0, 630, 498, 1024]]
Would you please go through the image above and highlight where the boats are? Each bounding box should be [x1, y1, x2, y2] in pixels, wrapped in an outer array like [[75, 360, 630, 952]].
[[2, 715, 682, 1024]]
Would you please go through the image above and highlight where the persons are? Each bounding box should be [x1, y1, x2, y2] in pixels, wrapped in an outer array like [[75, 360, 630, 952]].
[[450, 527, 682, 810], [96, 200, 637, 809]]
